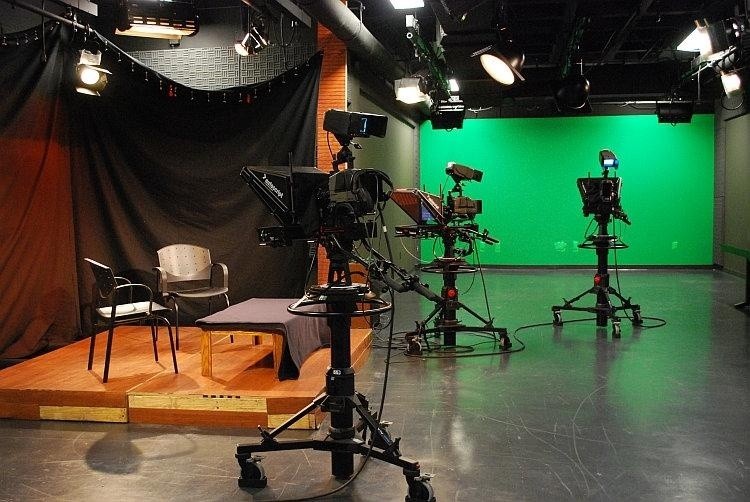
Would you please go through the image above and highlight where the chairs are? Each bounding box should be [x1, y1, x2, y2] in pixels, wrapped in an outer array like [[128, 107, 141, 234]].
[[84, 243, 233, 384]]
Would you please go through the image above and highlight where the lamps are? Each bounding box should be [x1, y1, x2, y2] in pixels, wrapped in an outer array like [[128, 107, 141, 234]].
[[673, 18, 748, 96], [75, 29, 111, 99], [392, 57, 431, 104], [115, 1, 200, 51], [234, 1, 274, 58], [478, 0, 527, 86]]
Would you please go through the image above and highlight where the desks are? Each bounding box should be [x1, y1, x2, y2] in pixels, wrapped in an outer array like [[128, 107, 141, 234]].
[[195, 298, 330, 382]]
